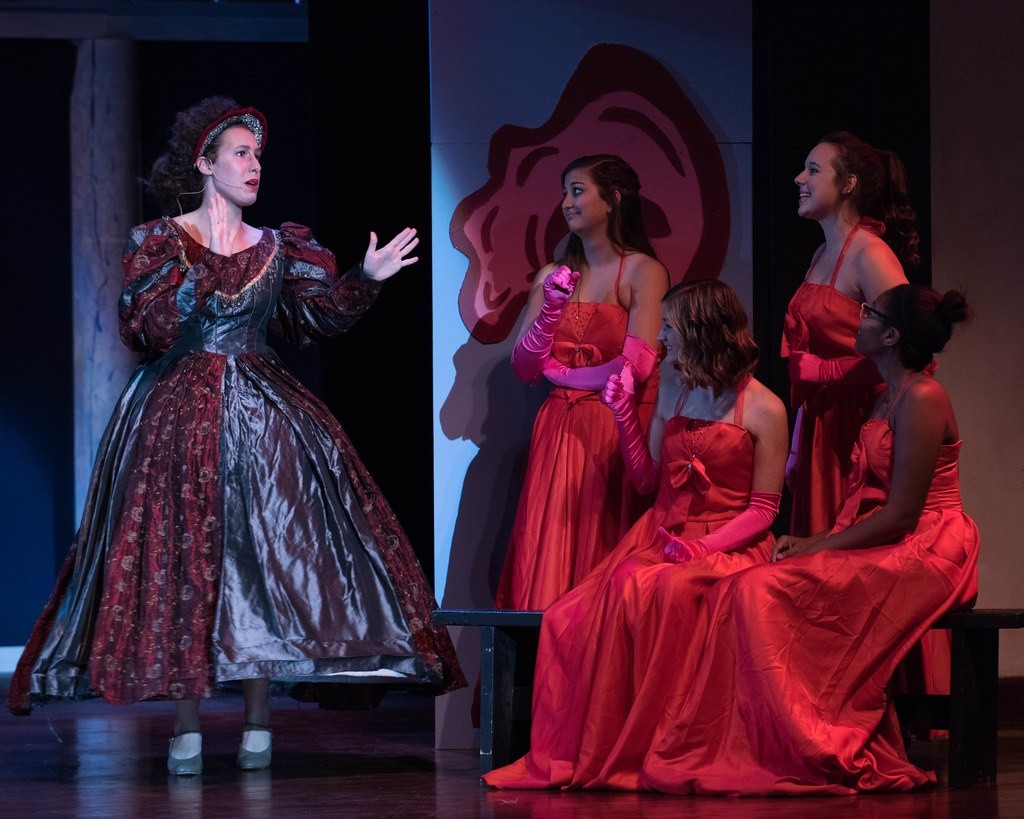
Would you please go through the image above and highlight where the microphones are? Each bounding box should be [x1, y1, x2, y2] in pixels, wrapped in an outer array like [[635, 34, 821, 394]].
[[209, 160, 243, 189]]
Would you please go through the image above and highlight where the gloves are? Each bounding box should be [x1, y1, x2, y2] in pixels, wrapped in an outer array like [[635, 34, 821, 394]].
[[787, 350, 938, 386], [658, 490, 782, 564], [529, 333, 659, 392], [599, 361, 662, 495], [510, 265, 581, 384]]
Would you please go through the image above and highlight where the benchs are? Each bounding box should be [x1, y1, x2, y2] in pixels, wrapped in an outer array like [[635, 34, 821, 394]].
[[427, 608, 1023, 786]]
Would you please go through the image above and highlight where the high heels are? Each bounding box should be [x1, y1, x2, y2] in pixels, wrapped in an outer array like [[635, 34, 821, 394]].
[[166, 730, 203, 775], [237, 722, 273, 770]]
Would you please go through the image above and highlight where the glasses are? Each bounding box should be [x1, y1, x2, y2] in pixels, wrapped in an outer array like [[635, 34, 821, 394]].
[[859, 302, 894, 323]]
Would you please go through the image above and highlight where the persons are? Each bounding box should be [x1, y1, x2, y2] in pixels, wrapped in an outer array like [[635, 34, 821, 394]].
[[493, 152, 672, 611], [490, 280, 981, 796], [779, 128, 928, 542], [0, 98, 469, 777]]
